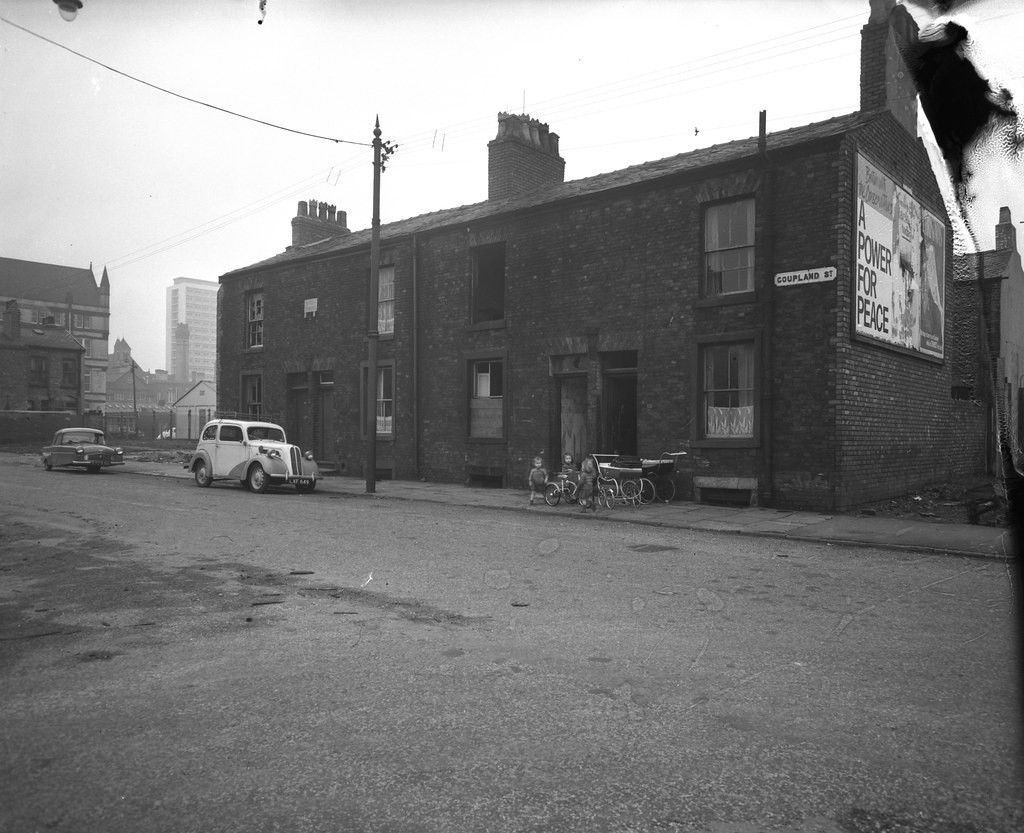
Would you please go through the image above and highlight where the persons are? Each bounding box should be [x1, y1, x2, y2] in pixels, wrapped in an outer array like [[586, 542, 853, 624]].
[[528, 457, 549, 505], [562, 453, 596, 513]]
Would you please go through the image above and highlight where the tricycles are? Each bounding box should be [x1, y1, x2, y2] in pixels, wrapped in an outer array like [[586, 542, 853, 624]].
[[543, 474, 594, 509]]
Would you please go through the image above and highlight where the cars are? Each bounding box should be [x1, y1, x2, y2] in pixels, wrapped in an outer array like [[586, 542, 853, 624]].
[[40, 428, 126, 474], [183, 419, 324, 494]]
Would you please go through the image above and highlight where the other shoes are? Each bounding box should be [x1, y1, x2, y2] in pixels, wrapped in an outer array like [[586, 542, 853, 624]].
[[529, 500, 532, 505], [581, 505, 587, 513], [591, 503, 596, 513]]
